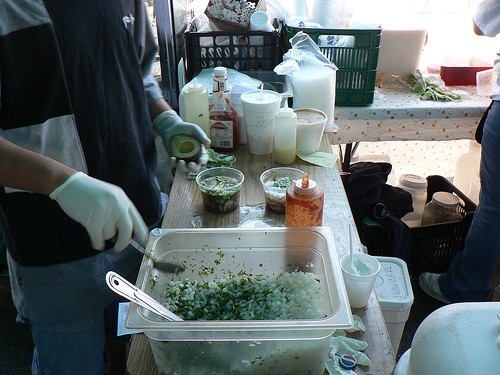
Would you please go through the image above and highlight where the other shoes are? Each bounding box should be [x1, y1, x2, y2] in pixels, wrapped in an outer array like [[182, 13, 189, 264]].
[[418, 273, 453, 304]]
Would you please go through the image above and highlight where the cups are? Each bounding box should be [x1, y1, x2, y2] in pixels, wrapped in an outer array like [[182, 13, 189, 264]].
[[240, 88, 282, 156], [341, 250, 380, 309], [249, 10, 275, 34]]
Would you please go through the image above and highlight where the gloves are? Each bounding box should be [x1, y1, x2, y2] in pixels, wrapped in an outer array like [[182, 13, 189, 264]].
[[324, 315, 373, 375], [152, 110, 211, 172], [50, 172, 149, 252]]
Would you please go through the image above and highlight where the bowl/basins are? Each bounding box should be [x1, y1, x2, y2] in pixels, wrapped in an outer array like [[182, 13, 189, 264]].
[[194, 167, 245, 214], [259, 167, 308, 212], [292, 106, 327, 155]]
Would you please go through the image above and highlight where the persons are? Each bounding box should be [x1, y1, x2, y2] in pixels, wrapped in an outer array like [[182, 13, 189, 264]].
[[417, 0, 500, 302], [0, 0, 212, 375]]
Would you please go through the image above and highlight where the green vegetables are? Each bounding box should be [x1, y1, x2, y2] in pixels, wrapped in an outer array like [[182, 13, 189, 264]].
[[189, 150, 234, 176]]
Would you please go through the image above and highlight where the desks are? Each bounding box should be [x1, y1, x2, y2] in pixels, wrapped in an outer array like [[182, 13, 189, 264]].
[[331, 86, 493, 172], [127, 132, 395, 375]]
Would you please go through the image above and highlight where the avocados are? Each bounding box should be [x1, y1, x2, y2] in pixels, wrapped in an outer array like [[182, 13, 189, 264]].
[[168, 133, 201, 162]]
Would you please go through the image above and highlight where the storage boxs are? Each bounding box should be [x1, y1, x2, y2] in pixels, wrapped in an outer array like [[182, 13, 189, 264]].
[[204, 0, 267, 30], [124, 227, 355, 375], [387, 175, 477, 274], [371, 255, 414, 356], [279, 22, 382, 105], [184, 13, 283, 93]]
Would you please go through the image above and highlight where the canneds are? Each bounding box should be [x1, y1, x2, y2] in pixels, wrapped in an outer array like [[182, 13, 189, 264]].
[[420, 192, 462, 224], [396, 174, 427, 227]]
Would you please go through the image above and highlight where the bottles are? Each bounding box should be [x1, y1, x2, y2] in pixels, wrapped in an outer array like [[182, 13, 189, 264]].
[[272, 100, 297, 165], [285, 173, 325, 253], [207, 67, 239, 152], [397, 172, 463, 230], [183, 77, 211, 144]]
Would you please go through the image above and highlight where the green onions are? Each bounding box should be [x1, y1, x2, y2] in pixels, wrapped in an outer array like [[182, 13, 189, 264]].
[[200, 173, 239, 203], [268, 175, 295, 189], [160, 250, 325, 321]]
